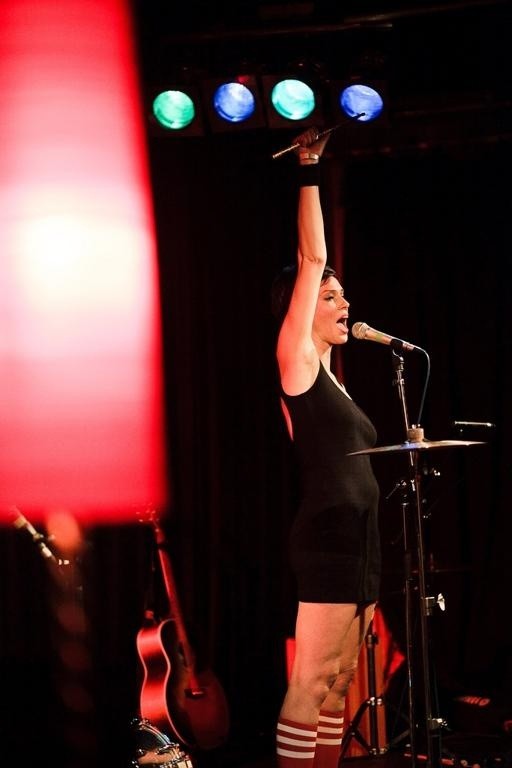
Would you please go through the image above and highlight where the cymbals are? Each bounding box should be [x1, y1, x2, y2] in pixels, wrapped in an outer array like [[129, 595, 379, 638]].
[[345, 439, 487, 456], [411, 563, 468, 574]]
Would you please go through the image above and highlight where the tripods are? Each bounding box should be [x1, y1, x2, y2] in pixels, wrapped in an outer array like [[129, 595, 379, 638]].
[[337, 628, 414, 767]]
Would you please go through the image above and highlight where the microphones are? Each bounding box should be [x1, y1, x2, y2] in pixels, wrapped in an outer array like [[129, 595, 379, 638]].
[[349, 319, 418, 357]]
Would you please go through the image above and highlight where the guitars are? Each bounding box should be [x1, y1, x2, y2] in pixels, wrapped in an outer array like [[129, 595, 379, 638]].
[[135, 504, 232, 752]]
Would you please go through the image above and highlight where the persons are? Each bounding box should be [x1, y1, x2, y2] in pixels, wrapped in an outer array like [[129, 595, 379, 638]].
[[267, 124, 382, 768]]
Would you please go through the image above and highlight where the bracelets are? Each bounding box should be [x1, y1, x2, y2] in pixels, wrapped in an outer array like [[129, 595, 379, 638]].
[[297, 165, 320, 188], [298, 151, 319, 161]]
